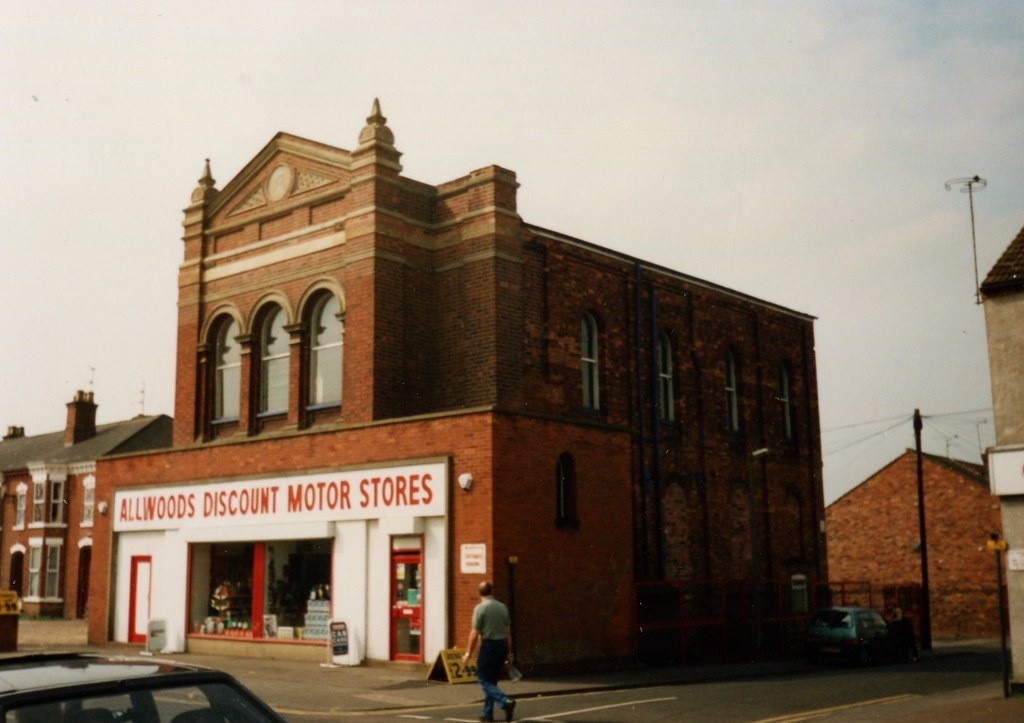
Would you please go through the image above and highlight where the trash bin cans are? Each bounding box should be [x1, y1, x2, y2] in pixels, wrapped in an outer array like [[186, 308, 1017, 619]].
[[887, 621, 909, 665]]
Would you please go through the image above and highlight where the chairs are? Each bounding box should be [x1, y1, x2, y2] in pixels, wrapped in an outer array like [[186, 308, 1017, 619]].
[[170, 708, 227, 723], [65, 708, 116, 723]]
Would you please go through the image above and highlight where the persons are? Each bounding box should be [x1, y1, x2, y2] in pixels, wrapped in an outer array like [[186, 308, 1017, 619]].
[[461, 580, 516, 723]]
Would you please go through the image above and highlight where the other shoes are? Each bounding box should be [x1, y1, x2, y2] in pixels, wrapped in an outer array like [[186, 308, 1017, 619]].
[[479, 716, 494, 722], [504, 700, 516, 723]]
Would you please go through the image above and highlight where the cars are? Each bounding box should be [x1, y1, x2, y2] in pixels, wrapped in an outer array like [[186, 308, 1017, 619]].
[[802, 605, 893, 670], [0, 652, 291, 723]]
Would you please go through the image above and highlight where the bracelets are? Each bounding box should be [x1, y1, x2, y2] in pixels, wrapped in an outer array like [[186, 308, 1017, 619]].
[[464, 652, 471, 658]]
